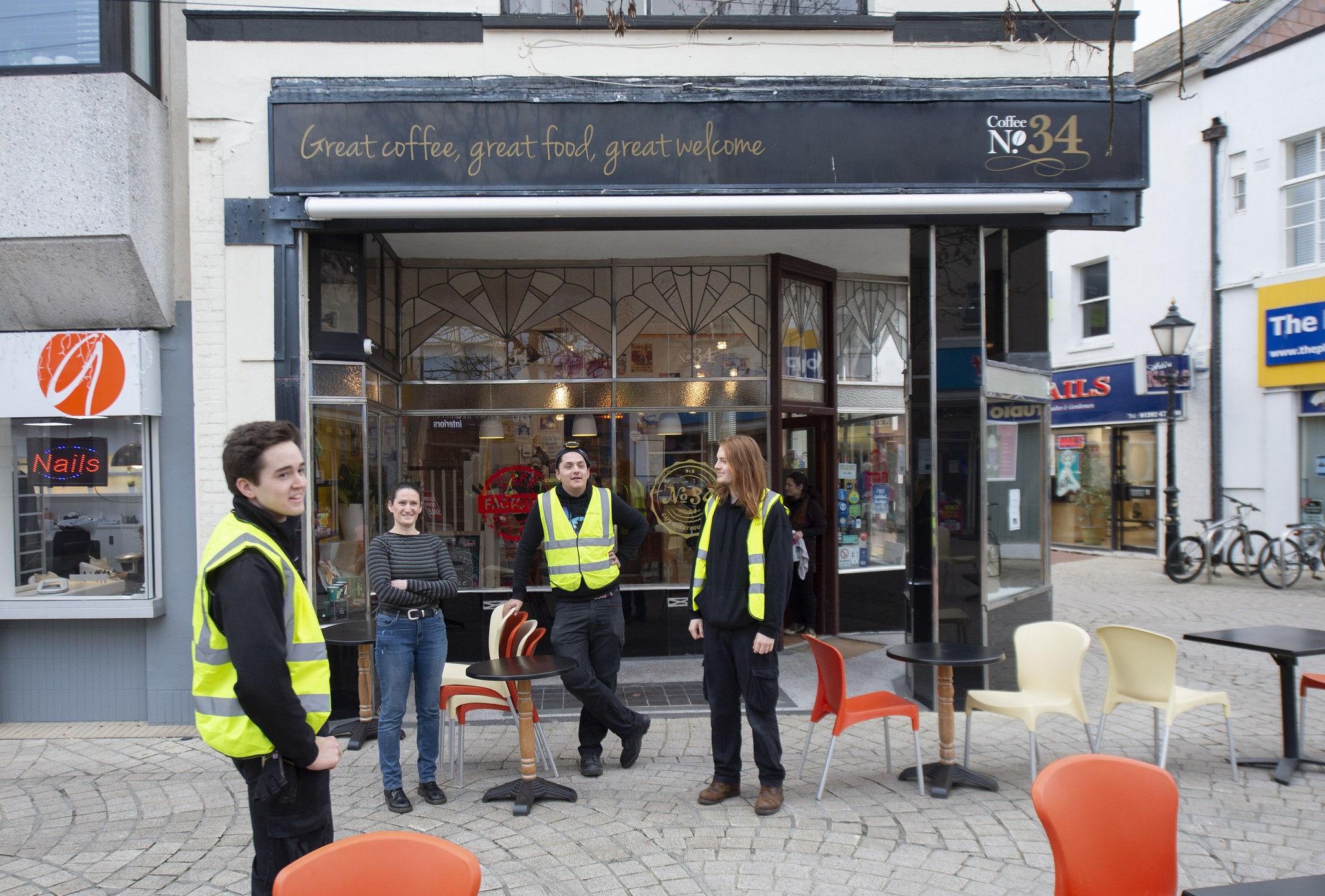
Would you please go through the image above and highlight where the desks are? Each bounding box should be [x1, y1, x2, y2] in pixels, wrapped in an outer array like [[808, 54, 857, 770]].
[[1181, 874, 1325, 896], [838, 564, 906, 574], [1182, 625, 1325, 786], [886, 643, 1006, 800], [15, 579, 125, 595], [322, 621, 406, 751], [465, 653, 580, 818], [457, 582, 699, 656]]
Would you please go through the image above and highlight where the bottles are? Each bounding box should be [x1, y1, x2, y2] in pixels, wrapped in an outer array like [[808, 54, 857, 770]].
[[45, 511, 51, 519]]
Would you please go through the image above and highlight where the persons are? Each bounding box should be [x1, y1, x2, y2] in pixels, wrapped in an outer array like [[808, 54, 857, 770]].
[[114, 526, 144, 583], [783, 472, 828, 639], [502, 441, 650, 777], [686, 435, 794, 815], [192, 421, 342, 895], [366, 482, 458, 814]]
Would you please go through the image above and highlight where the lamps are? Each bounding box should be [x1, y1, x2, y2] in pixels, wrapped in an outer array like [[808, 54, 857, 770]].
[[572, 330, 597, 436], [478, 331, 504, 439], [111, 430, 142, 472], [657, 319, 682, 435], [694, 314, 738, 377]]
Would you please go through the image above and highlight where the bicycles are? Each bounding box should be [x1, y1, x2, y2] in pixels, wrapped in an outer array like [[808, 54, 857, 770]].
[[1164, 494, 1274, 584], [987, 503, 1001, 576], [1258, 523, 1325, 590]]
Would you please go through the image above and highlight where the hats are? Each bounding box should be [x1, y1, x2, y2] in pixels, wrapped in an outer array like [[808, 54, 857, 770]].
[[555, 441, 591, 468]]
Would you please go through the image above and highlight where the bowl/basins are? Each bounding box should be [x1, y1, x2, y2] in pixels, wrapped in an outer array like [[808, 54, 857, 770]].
[[121, 516, 136, 524]]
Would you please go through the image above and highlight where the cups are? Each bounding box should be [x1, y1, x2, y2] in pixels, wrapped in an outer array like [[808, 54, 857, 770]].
[[335, 581, 349, 596], [331, 584, 347, 599], [327, 586, 342, 601]]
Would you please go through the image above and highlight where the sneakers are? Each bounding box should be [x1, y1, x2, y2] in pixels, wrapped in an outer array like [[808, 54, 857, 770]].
[[806, 628, 816, 638], [620, 714, 651, 769], [784, 622, 805, 634], [580, 752, 603, 776]]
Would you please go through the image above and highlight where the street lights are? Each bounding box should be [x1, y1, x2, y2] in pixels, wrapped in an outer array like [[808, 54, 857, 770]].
[[1151, 296, 1196, 574]]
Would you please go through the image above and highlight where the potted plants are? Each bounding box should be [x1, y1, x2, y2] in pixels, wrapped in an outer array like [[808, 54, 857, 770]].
[[127, 480, 136, 493]]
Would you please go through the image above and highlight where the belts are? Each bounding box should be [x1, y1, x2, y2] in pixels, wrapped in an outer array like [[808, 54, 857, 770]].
[[378, 602, 440, 621]]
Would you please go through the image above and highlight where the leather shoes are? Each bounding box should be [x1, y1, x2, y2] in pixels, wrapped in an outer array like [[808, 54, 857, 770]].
[[384, 787, 413, 813], [417, 782, 447, 805], [754, 785, 784, 815], [697, 781, 741, 805]]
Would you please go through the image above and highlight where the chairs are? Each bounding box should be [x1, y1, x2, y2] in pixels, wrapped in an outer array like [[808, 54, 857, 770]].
[[439, 603, 560, 787], [272, 831, 481, 896], [1299, 674, 1325, 755], [796, 634, 926, 800], [964, 620, 1238, 896]]
